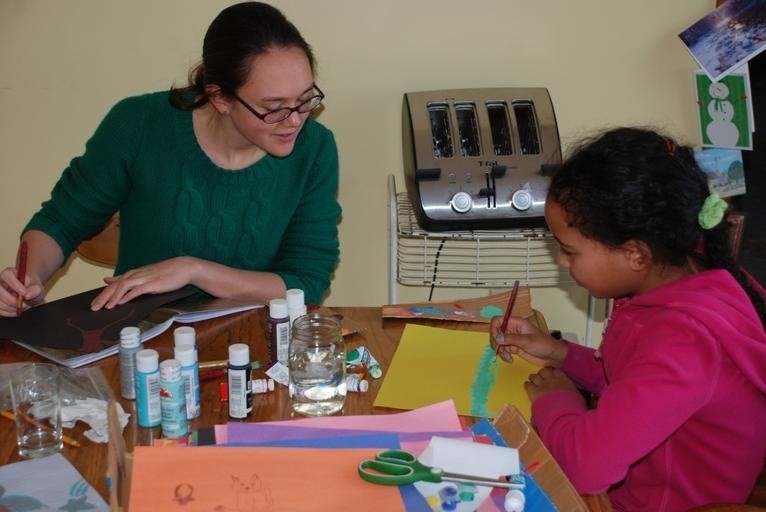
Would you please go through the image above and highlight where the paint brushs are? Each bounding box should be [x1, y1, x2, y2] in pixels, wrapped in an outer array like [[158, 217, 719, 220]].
[[16, 407, 81, 449], [17, 241, 28, 319], [198, 360, 264, 380], [1, 409, 18, 420], [492, 279, 520, 362]]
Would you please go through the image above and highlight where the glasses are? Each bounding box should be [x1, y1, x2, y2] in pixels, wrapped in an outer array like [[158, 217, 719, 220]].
[[236, 84, 326, 124]]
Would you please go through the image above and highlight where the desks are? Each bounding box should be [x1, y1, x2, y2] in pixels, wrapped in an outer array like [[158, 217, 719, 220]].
[[0, 307, 614, 512]]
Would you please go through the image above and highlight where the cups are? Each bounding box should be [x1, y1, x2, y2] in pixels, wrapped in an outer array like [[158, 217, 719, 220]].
[[8, 364, 63, 458]]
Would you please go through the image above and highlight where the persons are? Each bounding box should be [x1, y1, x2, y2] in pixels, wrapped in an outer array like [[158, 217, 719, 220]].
[[0, 3, 348, 321], [488, 124, 765, 512]]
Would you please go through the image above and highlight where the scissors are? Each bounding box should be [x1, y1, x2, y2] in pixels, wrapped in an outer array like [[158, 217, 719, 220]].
[[359, 450, 527, 489]]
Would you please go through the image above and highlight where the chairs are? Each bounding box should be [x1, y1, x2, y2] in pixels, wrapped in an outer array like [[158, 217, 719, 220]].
[[73, 213, 122, 266]]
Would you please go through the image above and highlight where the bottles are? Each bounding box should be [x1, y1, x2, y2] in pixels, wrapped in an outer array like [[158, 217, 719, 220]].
[[119, 327, 203, 438], [268, 289, 347, 417], [227, 343, 252, 420]]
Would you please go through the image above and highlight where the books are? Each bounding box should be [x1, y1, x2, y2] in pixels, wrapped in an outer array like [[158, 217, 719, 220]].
[[0, 285, 266, 371]]
